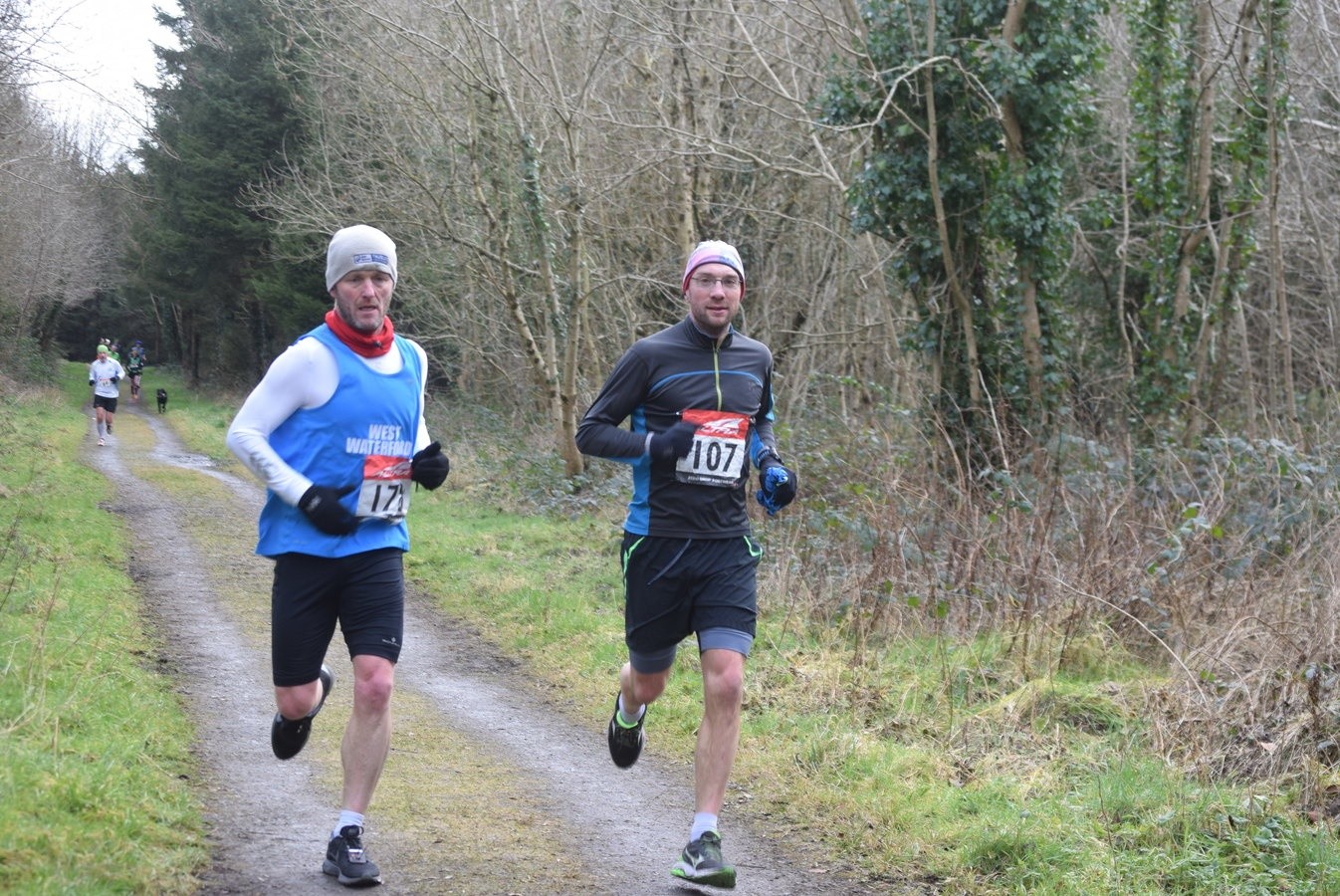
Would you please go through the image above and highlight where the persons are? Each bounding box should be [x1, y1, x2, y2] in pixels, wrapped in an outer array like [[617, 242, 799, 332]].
[[227, 224, 448, 889], [573, 244, 798, 889], [89, 337, 147, 447]]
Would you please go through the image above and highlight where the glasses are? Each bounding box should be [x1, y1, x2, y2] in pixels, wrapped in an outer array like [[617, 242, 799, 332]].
[[691, 275, 742, 289]]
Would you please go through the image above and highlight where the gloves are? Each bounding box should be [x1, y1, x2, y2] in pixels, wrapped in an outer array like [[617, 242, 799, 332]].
[[297, 484, 360, 536], [757, 456, 798, 516], [89, 380, 94, 386], [650, 421, 700, 462], [411, 441, 449, 490], [111, 378, 116, 383]]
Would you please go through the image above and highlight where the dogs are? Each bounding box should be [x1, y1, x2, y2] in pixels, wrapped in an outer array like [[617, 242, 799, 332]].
[[157, 387, 167, 414]]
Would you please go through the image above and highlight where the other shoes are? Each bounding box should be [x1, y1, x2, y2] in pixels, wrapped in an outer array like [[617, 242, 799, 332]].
[[131, 395, 137, 399], [106, 422, 111, 434], [97, 438, 105, 446]]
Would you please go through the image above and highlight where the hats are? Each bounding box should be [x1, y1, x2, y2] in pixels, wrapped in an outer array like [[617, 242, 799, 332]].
[[97, 344, 108, 353], [681, 239, 746, 300]]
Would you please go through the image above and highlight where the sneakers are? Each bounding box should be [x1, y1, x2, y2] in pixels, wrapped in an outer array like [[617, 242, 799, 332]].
[[322, 823, 382, 886], [671, 830, 736, 888], [270, 665, 331, 759], [607, 690, 648, 768]]
